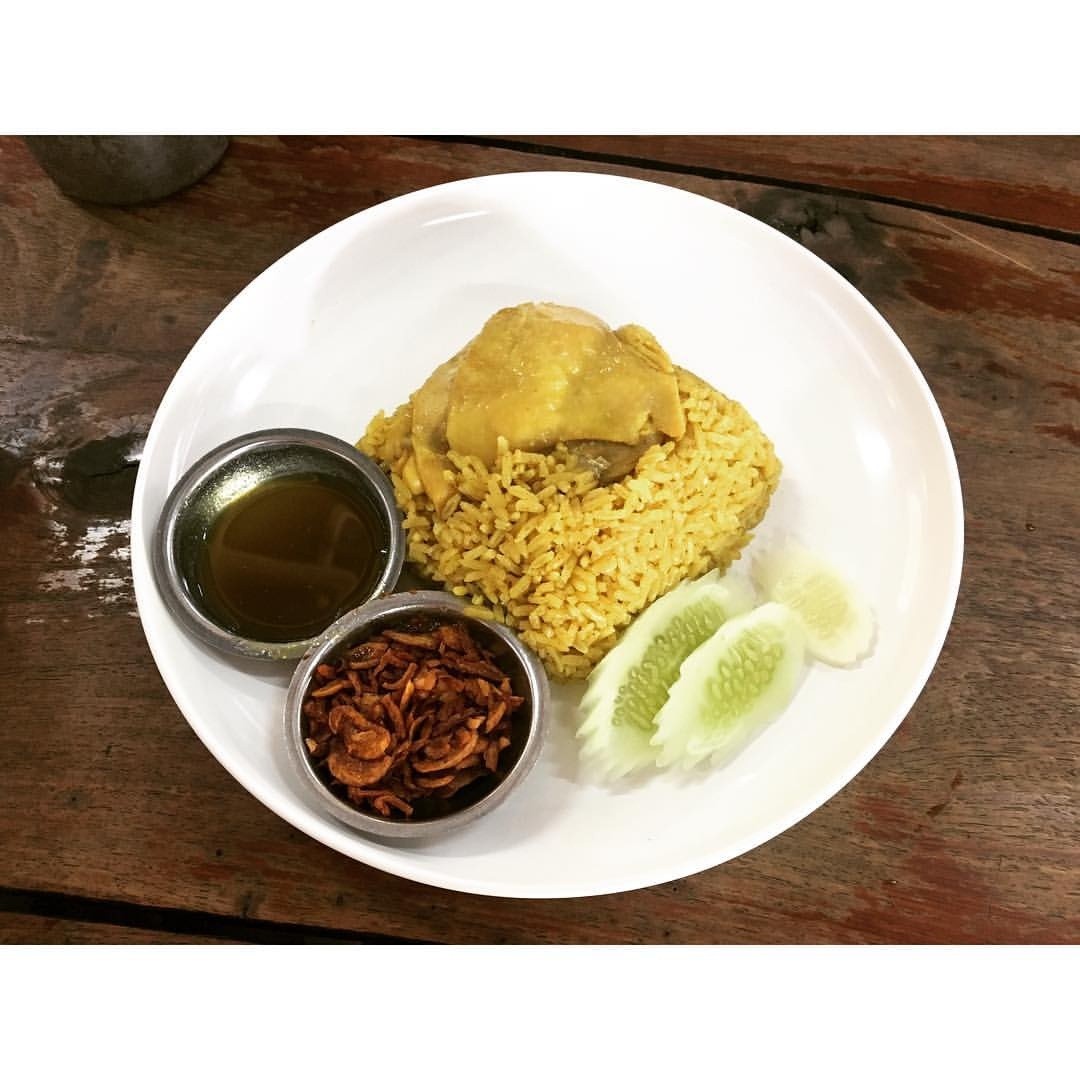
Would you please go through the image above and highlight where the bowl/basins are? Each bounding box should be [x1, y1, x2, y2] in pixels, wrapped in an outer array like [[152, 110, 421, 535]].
[[284, 591, 551, 839], [151, 426, 407, 664]]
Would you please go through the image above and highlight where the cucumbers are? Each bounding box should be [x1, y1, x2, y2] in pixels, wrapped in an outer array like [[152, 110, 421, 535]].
[[568, 537, 875, 785]]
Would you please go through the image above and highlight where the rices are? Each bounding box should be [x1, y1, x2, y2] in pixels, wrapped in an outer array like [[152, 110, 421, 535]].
[[345, 345, 783, 689]]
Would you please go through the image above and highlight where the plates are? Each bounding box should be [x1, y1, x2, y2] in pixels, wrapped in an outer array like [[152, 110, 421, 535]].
[[126, 169, 968, 900]]
